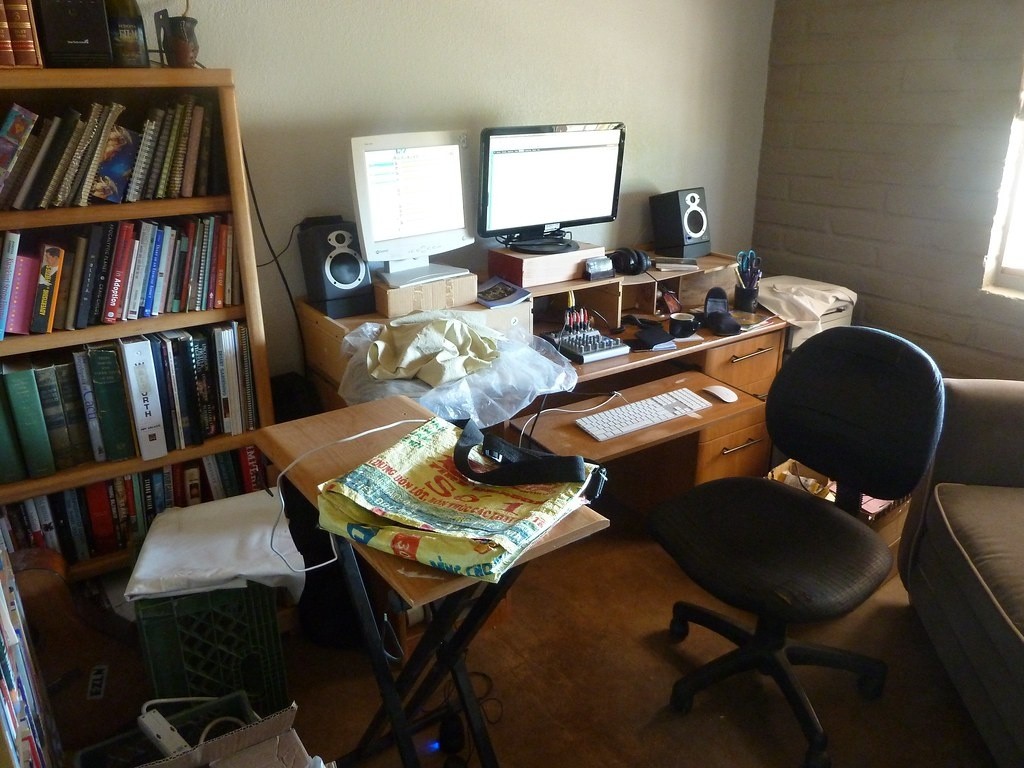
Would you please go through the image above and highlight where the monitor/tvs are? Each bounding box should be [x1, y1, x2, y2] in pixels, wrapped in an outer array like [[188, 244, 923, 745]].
[[478, 121, 626, 254], [349, 129, 475, 289]]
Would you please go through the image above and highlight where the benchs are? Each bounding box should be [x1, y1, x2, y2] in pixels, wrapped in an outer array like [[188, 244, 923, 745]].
[[511, 370, 765, 465]]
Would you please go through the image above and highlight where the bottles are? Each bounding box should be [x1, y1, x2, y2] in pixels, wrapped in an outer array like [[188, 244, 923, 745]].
[[661, 284, 681, 313]]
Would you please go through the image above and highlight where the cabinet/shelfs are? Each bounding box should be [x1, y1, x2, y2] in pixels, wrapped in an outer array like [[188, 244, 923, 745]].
[[0, 63, 299, 750], [295, 243, 744, 383]]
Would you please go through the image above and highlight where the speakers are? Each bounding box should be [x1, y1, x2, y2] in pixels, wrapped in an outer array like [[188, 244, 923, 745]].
[[649, 187, 711, 260], [297, 222, 377, 320]]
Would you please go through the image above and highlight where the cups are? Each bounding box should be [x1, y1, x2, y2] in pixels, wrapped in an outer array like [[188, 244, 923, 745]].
[[669, 313, 702, 338], [734, 284, 759, 313]]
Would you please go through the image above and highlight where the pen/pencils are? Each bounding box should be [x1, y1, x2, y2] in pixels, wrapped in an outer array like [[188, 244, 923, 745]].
[[733, 263, 763, 289]]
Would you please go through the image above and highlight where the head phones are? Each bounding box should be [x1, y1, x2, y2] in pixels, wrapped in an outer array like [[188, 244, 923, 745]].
[[605, 247, 652, 277]]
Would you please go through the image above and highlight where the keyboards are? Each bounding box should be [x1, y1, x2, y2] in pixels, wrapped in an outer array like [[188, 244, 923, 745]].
[[573, 388, 712, 441]]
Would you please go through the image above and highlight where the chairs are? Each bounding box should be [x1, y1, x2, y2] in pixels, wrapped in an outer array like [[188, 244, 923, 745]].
[[652, 326, 946, 768]]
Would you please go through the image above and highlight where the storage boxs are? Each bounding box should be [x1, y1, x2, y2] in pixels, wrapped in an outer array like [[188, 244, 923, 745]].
[[371, 273, 478, 318], [73, 690, 262, 768], [140, 701, 338, 768], [127, 536, 289, 717]]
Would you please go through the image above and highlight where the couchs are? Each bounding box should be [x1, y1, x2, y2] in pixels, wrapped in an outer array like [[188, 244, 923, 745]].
[[898, 377, 1024, 768]]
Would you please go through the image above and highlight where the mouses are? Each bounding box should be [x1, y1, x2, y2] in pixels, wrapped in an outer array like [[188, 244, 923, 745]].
[[703, 385, 738, 402]]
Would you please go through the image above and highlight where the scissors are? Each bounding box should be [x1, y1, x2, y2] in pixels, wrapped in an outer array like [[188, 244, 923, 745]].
[[734, 251, 759, 269]]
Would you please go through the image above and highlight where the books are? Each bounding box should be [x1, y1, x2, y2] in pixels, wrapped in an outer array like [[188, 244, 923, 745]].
[[477, 275, 532, 310], [0, 0, 269, 768]]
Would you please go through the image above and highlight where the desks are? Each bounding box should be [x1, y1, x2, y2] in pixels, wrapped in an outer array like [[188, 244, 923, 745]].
[[294, 272, 856, 498], [248, 395, 610, 768]]
